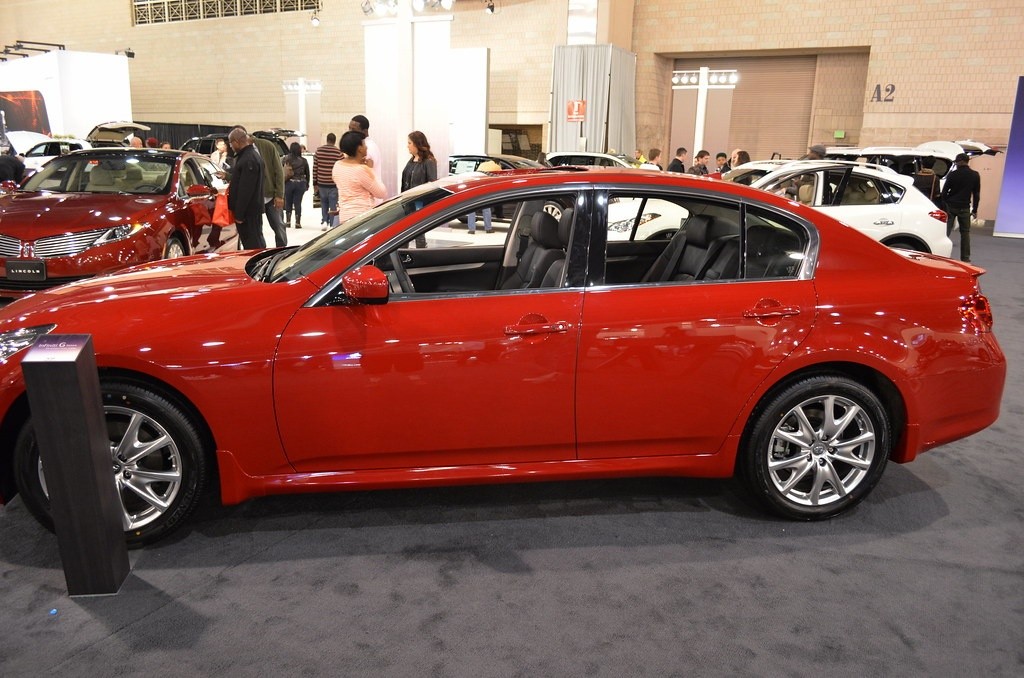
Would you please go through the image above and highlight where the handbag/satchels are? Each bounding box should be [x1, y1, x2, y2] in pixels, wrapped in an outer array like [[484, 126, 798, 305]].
[[313, 191, 321, 208], [211, 187, 235, 228], [283, 155, 295, 182]]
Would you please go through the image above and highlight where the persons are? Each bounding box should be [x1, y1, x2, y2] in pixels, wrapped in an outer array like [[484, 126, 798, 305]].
[[640, 149, 663, 171], [715, 152, 727, 172], [632, 148, 647, 168], [228, 125, 288, 247], [913, 155, 940, 202], [538, 152, 549, 167], [667, 147, 688, 173], [313, 133, 345, 231], [211, 139, 234, 172], [281, 142, 310, 228], [782, 145, 830, 204], [468, 158, 503, 234], [721, 148, 752, 186], [214, 128, 266, 250], [688, 150, 710, 176], [332, 130, 388, 223], [941, 153, 981, 263], [131, 137, 170, 149], [401, 131, 438, 248], [349, 115, 384, 204], [0, 152, 25, 184]]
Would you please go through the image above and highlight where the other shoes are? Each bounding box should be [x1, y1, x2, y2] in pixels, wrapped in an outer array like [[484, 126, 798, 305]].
[[468, 231, 476, 233], [322, 221, 327, 231], [486, 230, 494, 232]]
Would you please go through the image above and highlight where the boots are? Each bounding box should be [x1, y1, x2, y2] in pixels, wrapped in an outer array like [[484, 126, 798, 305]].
[[286, 212, 291, 227], [295, 214, 301, 227]]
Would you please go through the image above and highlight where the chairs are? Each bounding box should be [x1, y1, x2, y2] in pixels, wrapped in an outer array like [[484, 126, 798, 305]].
[[780, 177, 828, 201], [84, 166, 196, 191], [47, 143, 61, 156], [638, 214, 803, 277], [501, 206, 578, 288], [841, 183, 880, 203]]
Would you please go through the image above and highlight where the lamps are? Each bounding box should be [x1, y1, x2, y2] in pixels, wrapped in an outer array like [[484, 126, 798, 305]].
[[671, 72, 737, 84], [283, 83, 325, 91], [116, 48, 145, 57], [310, 9, 320, 26], [0, 40, 65, 62], [485, 0, 496, 14], [361, 0, 456, 15]]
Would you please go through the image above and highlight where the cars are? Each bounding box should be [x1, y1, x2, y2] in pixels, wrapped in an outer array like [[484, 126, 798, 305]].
[[0, 119, 308, 196], [1, 161, 1010, 553], [1, 144, 230, 300], [409, 138, 1007, 259]]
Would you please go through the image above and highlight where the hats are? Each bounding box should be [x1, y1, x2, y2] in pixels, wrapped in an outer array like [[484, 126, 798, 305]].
[[808, 145, 826, 155], [952, 153, 969, 162]]
